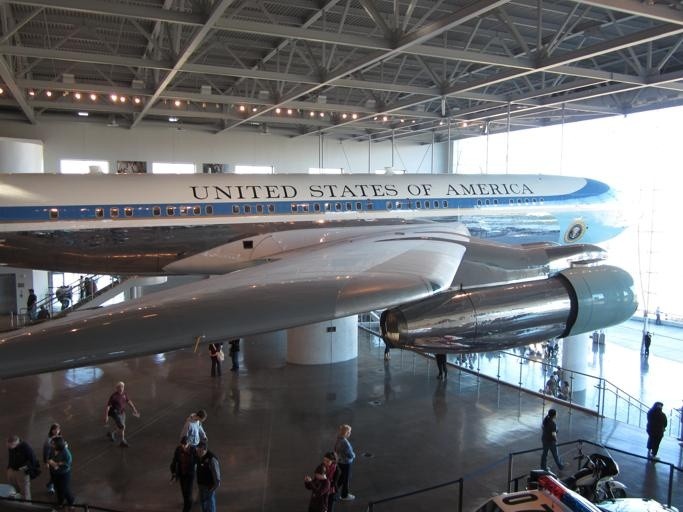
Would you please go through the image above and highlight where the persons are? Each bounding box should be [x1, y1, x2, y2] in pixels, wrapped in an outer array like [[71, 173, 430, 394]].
[[227, 338, 240, 371], [47, 435, 73, 507], [5, 433, 38, 500], [25, 275, 99, 321], [644, 331, 651, 354], [180, 409, 208, 473], [434, 351, 448, 380], [207, 341, 222, 378], [645, 400, 668, 457], [103, 381, 137, 447], [673, 399, 683, 441], [383, 342, 390, 360], [655, 306, 663, 325], [322, 451, 341, 512], [303, 463, 329, 512], [450, 337, 571, 399], [195, 442, 220, 512], [540, 407, 570, 471], [42, 422, 69, 495], [169, 435, 197, 511], [332, 423, 355, 500]]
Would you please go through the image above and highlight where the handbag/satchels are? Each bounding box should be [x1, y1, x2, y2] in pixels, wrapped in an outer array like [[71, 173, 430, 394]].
[[215, 351, 225, 364]]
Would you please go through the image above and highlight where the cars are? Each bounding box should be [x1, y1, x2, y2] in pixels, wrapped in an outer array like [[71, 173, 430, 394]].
[[471, 475, 678, 512]]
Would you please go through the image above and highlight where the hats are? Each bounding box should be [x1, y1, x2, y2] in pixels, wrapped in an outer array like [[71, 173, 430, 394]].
[[180, 435, 189, 445], [197, 442, 207, 449]]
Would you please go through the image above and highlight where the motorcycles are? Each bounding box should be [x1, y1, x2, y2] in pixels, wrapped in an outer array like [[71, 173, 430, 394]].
[[525, 438, 628, 504]]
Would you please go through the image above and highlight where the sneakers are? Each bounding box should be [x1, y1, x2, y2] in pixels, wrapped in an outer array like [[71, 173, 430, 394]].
[[48, 483, 56, 496], [106, 430, 115, 442], [120, 439, 130, 448], [340, 493, 355, 501]]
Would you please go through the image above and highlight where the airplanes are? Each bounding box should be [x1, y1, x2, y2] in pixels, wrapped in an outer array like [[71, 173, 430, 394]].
[[0, 173, 640, 380]]
